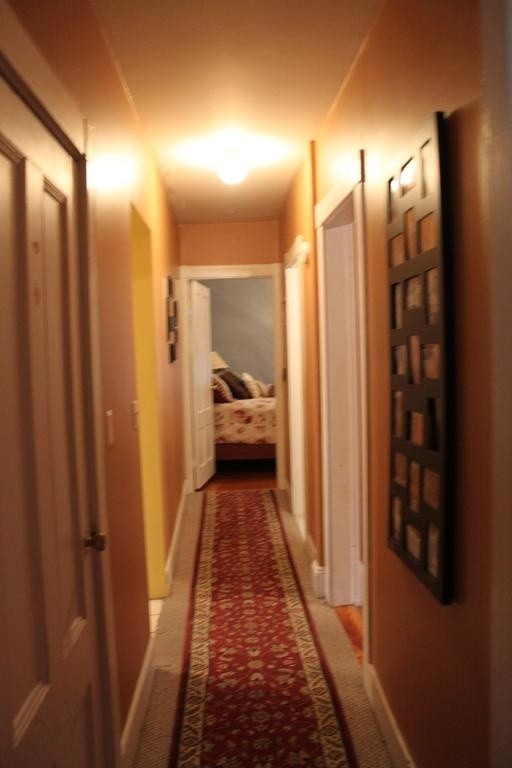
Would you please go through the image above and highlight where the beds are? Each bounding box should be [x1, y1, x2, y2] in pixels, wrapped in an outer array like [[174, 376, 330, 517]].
[[214, 397, 276, 461]]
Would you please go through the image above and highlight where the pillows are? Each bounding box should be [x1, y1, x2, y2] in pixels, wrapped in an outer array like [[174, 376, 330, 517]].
[[211, 370, 274, 403]]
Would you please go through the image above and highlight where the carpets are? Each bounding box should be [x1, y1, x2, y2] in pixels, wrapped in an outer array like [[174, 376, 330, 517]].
[[166, 487, 363, 767]]
